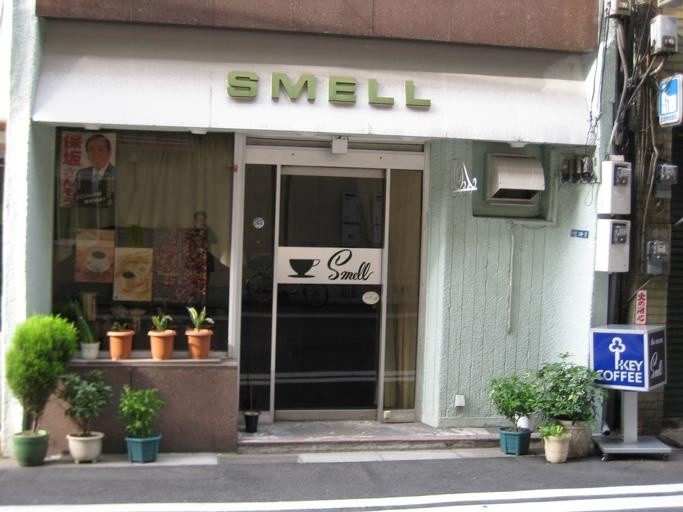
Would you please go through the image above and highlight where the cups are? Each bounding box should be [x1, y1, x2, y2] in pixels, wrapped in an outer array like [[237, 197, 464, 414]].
[[120, 271, 137, 291], [90, 250, 107, 269]]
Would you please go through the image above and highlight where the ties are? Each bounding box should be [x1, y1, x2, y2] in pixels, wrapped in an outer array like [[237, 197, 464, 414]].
[[93, 172, 101, 194]]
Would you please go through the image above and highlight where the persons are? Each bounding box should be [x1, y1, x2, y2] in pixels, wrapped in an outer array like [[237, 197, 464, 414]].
[[71, 133, 115, 209], [183, 209, 218, 309]]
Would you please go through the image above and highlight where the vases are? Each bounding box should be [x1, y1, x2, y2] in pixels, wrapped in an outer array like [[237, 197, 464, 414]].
[[245, 411, 260, 431]]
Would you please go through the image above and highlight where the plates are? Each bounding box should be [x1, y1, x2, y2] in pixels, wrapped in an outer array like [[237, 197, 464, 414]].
[[85, 257, 110, 273]]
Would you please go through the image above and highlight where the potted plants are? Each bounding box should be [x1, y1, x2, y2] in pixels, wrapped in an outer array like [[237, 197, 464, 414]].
[[489, 351, 611, 463], [5, 294, 217, 467]]
